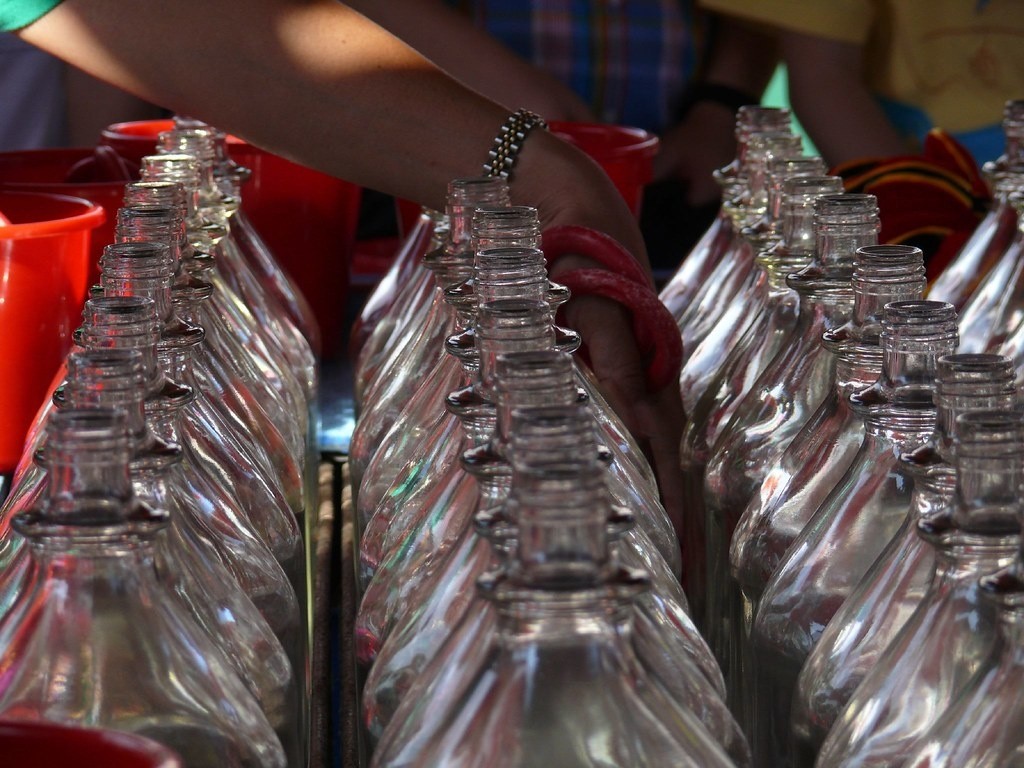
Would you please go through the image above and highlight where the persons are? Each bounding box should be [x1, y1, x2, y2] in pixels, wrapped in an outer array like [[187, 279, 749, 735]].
[[0, 0, 1024, 546]]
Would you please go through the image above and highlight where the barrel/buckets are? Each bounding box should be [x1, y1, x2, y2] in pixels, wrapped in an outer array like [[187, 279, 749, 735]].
[[397, 123, 664, 237], [0, 192, 106, 477], [101, 119, 364, 353], [1, 149, 139, 273]]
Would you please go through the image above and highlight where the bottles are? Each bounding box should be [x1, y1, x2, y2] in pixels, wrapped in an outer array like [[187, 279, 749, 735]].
[[0, 97, 1024, 768]]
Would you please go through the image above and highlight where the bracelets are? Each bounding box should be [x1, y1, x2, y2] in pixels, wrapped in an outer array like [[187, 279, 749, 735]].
[[481, 107, 549, 183], [684, 79, 761, 112]]
